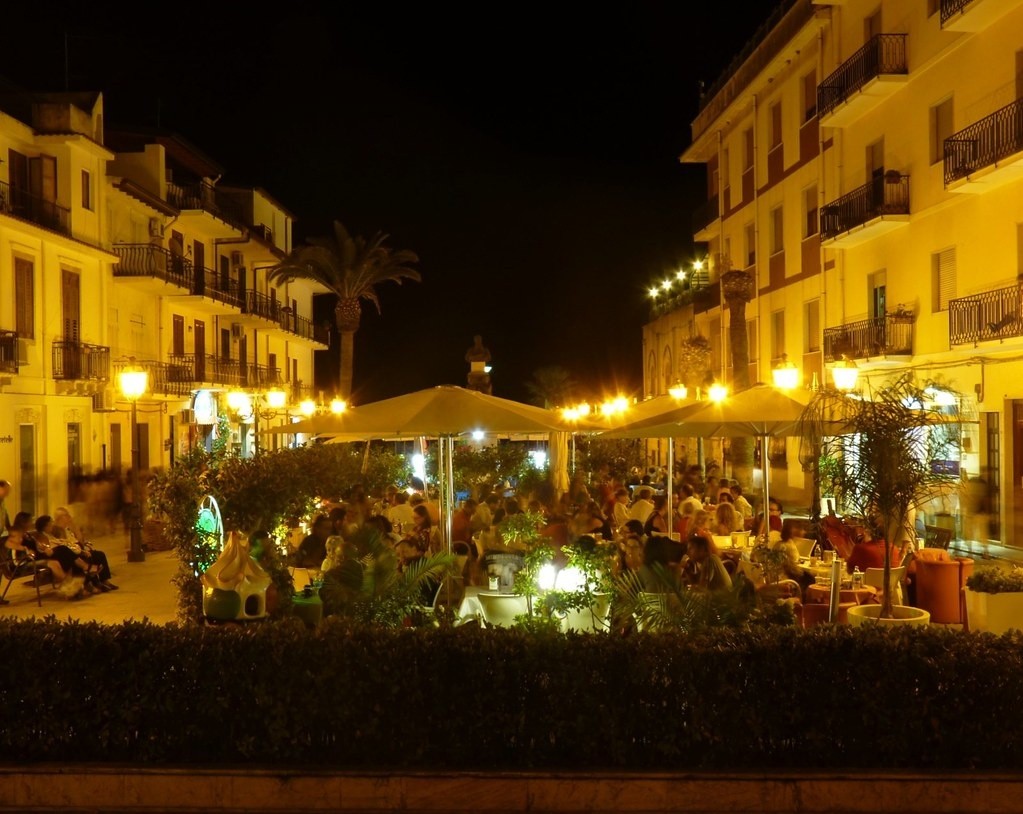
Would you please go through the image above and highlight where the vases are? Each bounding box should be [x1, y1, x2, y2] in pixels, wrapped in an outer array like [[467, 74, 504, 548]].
[[566, 590, 610, 634], [477, 589, 528, 629]]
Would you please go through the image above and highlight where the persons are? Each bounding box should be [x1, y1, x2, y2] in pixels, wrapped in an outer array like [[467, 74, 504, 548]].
[[245, 463, 815, 616], [844, 517, 900, 574], [121, 470, 132, 533], [876, 492, 916, 554], [7, 508, 120, 599], [0, 480, 12, 603]]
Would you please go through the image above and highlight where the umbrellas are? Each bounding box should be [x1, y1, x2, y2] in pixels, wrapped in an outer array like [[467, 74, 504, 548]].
[[249, 381, 982, 606]]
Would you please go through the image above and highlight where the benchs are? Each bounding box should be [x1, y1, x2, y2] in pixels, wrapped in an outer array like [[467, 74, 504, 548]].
[[0, 526, 64, 607]]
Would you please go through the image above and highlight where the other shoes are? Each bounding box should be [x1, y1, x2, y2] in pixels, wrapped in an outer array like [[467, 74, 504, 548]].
[[0, 597, 9, 605], [74, 581, 119, 600]]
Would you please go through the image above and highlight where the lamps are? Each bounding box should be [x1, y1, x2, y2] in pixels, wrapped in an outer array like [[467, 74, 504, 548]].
[[119, 352, 147, 398], [181, 390, 216, 425]]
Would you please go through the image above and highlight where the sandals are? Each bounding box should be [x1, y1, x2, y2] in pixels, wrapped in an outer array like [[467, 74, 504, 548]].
[[83, 564, 103, 577]]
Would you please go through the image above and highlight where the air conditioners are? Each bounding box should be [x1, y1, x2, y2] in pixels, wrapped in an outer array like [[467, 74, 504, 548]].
[[150, 216, 164, 239], [233, 323, 244, 338], [233, 250, 245, 268]]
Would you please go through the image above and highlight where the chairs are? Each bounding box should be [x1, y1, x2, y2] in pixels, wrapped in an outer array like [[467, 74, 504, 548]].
[[283, 486, 975, 630]]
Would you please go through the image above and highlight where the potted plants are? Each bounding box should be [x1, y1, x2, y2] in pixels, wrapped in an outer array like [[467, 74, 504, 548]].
[[960, 561, 1023, 642], [791, 353, 968, 633]]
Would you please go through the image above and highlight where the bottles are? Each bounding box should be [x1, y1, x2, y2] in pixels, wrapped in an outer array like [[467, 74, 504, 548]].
[[814, 544, 822, 563], [488, 567, 501, 591], [852, 566, 861, 590]]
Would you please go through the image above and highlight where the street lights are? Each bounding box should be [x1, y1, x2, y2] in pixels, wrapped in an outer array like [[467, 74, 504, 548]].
[[117, 353, 151, 562], [828, 359, 861, 513]]
